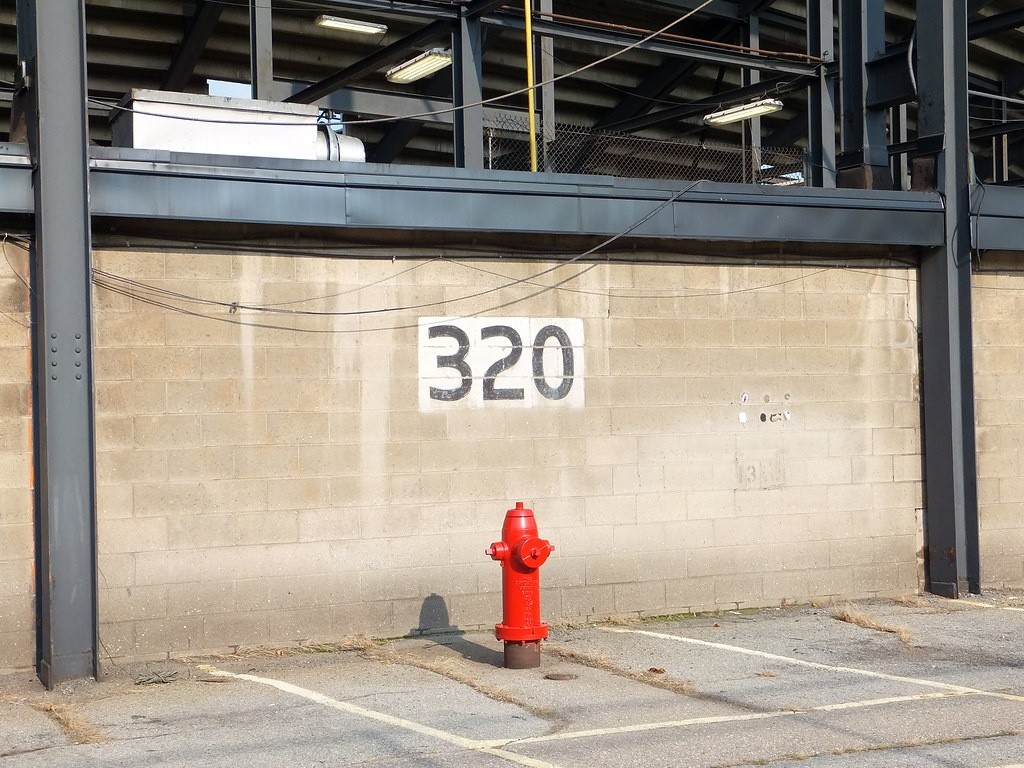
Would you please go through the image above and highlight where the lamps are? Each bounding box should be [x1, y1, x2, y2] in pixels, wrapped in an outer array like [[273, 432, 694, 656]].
[[385, 48, 452, 84], [702, 98, 784, 127], [312, 14, 389, 36]]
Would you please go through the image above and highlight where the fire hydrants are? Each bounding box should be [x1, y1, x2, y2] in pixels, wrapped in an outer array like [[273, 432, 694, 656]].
[[485, 502, 556, 669]]
